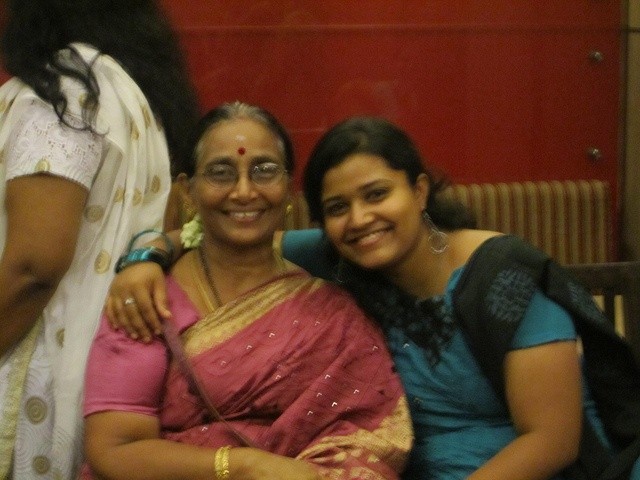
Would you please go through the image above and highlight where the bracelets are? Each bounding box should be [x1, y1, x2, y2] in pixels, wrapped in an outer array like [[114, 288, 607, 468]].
[[214, 444, 231, 480], [114, 248, 171, 274], [127, 228, 175, 257]]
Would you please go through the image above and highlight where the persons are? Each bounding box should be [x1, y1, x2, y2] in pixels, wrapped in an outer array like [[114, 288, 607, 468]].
[[102, 114, 639, 480], [75, 99, 414, 480], [0, 0, 199, 480]]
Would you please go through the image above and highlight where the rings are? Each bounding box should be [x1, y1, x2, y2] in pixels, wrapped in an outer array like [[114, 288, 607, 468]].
[[124, 297, 136, 304]]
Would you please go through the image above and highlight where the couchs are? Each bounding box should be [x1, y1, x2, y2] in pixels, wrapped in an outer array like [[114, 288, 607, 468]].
[[180, 181, 639, 341]]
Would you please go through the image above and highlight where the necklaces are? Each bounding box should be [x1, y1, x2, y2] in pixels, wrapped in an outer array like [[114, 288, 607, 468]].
[[193, 242, 287, 313]]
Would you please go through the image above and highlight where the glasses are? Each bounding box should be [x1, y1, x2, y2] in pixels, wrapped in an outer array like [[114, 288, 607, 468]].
[[194, 162, 291, 189]]
